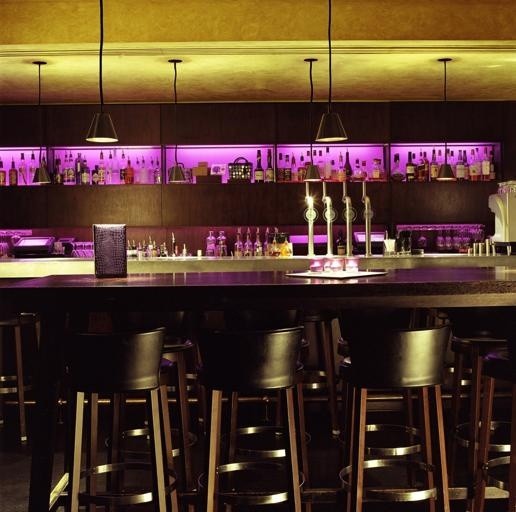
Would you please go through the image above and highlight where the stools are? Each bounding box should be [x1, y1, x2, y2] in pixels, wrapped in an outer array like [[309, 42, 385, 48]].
[[0, 310, 516, 511]]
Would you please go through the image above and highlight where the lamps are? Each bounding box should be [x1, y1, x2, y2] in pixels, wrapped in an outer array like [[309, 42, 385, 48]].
[[315, 0, 348, 142], [168, 59, 185, 182], [436, 58, 456, 181], [304, 58, 321, 182], [84, 0, 118, 143], [32, 61, 51, 183]]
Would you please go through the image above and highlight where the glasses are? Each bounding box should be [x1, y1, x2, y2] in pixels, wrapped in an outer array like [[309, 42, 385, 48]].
[[84, 0, 118, 143], [168, 59, 185, 182], [436, 58, 456, 181], [315, 0, 348, 142], [32, 61, 51, 183], [304, 58, 321, 182]]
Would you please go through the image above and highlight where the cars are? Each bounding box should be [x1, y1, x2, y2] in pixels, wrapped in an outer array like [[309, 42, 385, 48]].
[[310, 255, 359, 273]]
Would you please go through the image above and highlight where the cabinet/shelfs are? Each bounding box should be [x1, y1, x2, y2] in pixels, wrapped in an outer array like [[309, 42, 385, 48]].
[[382, 224, 497, 257], [336, 230, 346, 255], [127, 225, 293, 258]]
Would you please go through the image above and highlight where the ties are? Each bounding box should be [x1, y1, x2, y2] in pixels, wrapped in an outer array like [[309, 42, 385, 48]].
[[14, 237, 55, 249]]
